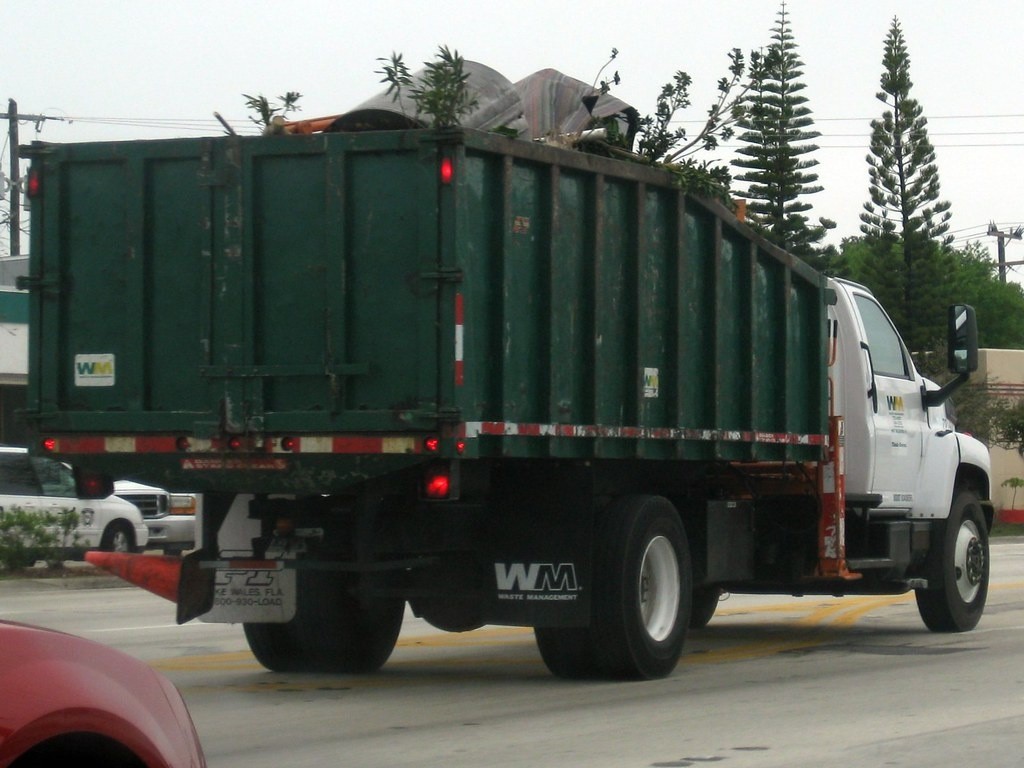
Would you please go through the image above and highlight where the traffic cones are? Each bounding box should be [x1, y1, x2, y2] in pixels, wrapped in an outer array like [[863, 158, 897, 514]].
[[85, 545, 217, 622]]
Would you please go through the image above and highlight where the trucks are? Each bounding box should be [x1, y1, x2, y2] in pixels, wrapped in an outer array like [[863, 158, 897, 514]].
[[0, 98, 996, 691]]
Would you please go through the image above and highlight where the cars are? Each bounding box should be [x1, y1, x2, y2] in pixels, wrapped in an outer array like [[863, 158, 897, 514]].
[[110, 477, 197, 556], [1, 620, 205, 768], [1, 446, 150, 562]]
